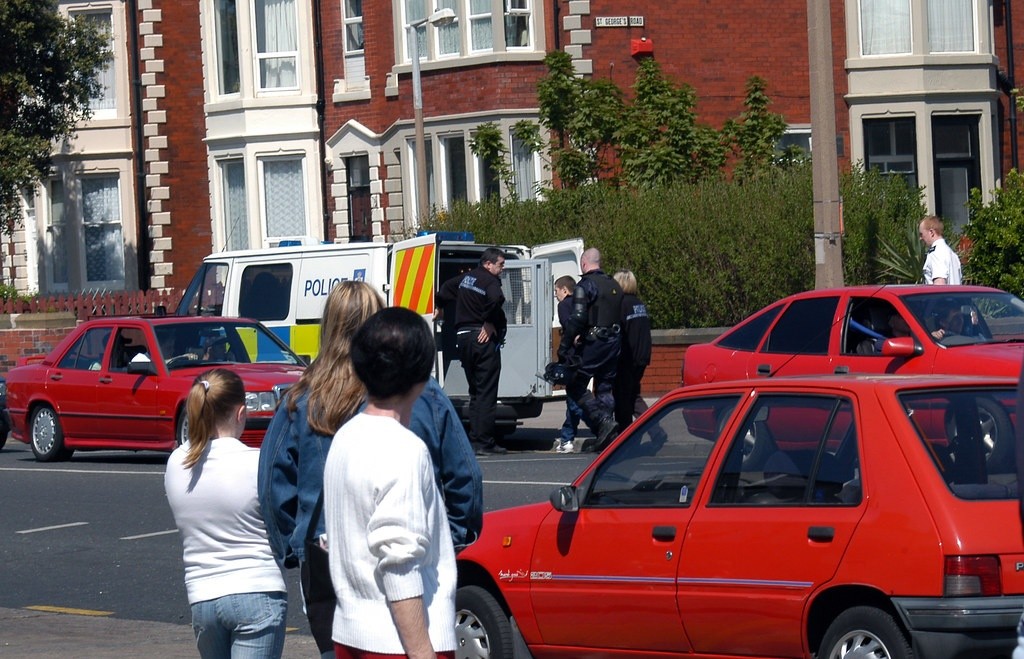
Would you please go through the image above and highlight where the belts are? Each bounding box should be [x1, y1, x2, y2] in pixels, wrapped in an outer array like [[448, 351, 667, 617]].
[[458, 326, 482, 331]]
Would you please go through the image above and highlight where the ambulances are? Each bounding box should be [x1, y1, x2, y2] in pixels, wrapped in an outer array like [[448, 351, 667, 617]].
[[154, 216, 594, 452]]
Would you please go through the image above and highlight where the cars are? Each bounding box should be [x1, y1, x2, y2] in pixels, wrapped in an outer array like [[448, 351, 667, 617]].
[[452, 373, 1024, 658], [679, 283, 1024, 474], [6, 316, 308, 463]]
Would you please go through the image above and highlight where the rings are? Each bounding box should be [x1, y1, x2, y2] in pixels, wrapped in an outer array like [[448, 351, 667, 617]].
[[191, 356, 193, 358]]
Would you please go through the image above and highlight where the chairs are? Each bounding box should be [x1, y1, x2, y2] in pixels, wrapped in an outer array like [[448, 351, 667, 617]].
[[744, 448, 844, 506]]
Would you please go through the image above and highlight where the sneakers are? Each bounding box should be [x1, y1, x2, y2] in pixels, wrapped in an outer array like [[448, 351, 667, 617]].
[[554, 436, 574, 453]]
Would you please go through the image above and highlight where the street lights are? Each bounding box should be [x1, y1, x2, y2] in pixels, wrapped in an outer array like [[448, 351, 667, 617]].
[[409, 6, 457, 232]]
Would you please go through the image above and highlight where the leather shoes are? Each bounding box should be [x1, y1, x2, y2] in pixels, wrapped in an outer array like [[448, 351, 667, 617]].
[[616, 442, 642, 458], [471, 441, 506, 457], [581, 417, 619, 453], [643, 428, 668, 456]]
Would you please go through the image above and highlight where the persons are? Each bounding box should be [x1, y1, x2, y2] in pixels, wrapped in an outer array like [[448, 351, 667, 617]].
[[103, 333, 132, 370], [876, 306, 946, 355], [259, 278, 484, 658], [433, 248, 509, 455], [567, 248, 622, 453], [932, 299, 969, 335], [131, 334, 199, 366], [551, 276, 589, 454], [612, 271, 667, 454], [204, 335, 237, 364], [319, 306, 461, 658], [918, 218, 963, 286], [164, 369, 287, 658]]
